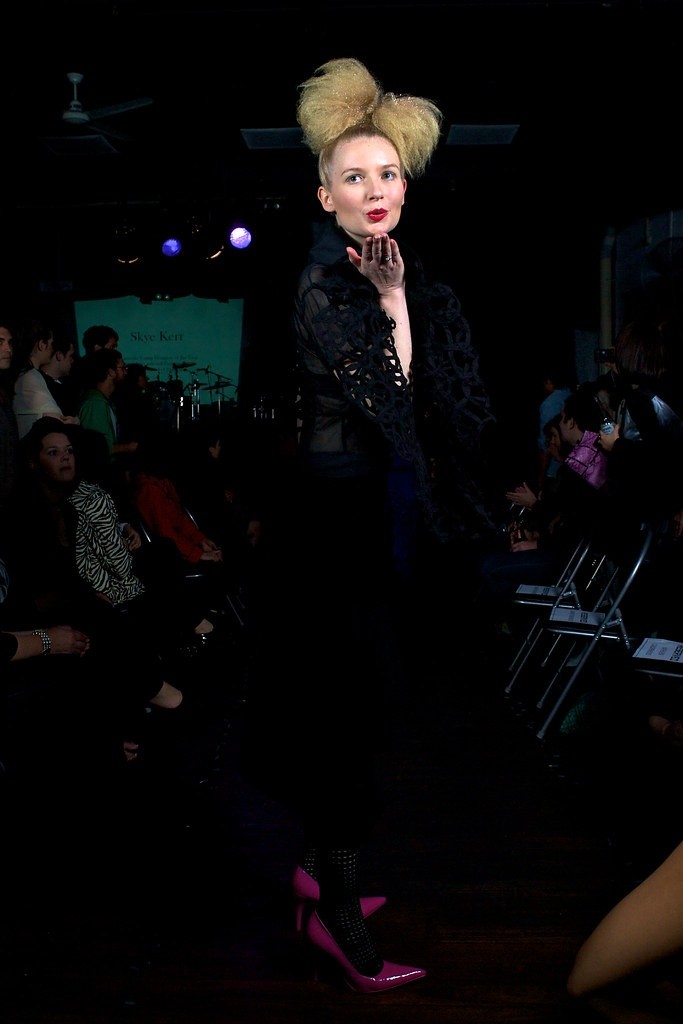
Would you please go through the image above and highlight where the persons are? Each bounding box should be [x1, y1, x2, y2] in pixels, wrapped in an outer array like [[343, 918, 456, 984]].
[[0, 322, 268, 831], [279, 58, 492, 993], [470, 318, 683, 748]]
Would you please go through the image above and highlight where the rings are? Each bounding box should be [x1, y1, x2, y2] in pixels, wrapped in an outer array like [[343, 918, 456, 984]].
[[383, 256, 393, 262]]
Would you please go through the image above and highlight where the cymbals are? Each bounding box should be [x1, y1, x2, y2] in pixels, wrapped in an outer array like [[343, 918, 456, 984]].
[[143, 364, 158, 371], [174, 362, 196, 368], [201, 381, 235, 390]]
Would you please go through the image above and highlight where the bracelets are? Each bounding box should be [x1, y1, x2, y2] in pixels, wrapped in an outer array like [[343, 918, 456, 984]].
[[32, 629, 51, 657]]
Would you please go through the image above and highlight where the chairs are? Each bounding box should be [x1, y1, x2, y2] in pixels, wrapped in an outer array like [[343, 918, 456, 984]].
[[504, 523, 683, 739], [140, 506, 245, 627]]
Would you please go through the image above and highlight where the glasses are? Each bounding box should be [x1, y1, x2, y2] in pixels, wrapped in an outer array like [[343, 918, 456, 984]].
[[112, 365, 129, 369]]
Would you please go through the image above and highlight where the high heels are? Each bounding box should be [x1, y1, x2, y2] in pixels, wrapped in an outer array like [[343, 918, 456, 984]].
[[294, 865, 387, 932], [307, 910, 428, 993]]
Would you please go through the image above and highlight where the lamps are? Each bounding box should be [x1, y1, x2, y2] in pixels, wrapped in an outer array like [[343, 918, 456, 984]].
[[111, 204, 144, 263], [192, 216, 224, 258]]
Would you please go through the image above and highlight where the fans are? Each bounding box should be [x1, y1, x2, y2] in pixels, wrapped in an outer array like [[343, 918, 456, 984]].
[[62, 73, 154, 141]]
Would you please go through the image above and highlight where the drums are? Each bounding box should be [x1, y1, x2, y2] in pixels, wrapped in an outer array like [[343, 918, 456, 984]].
[[171, 396, 192, 420]]
